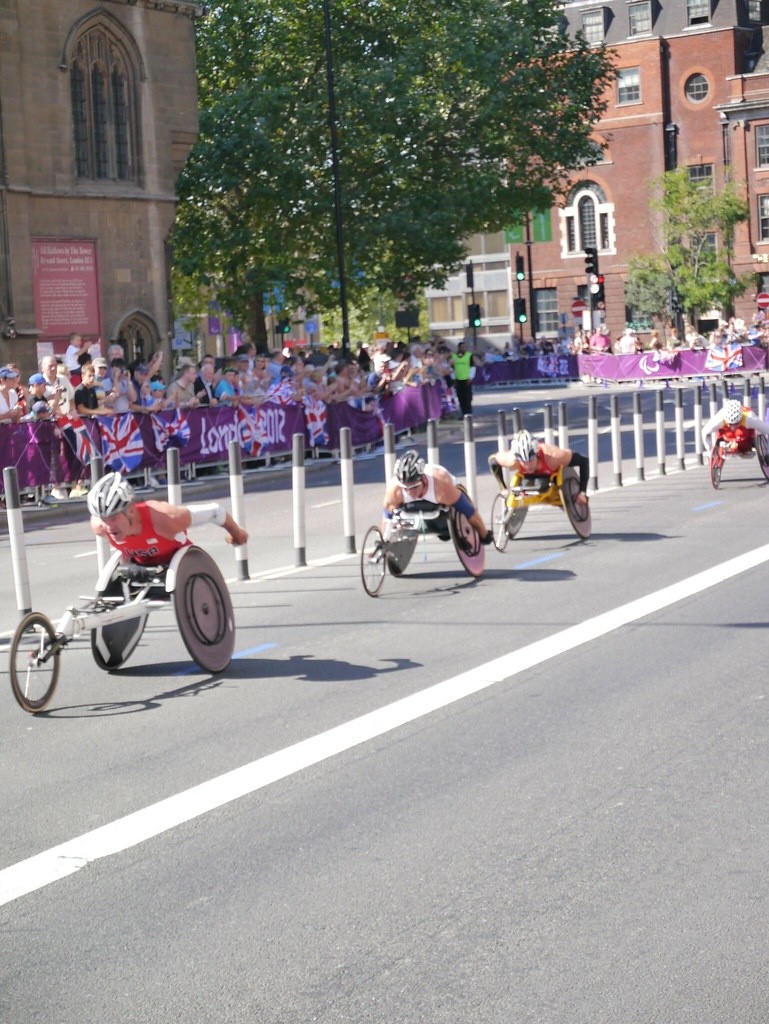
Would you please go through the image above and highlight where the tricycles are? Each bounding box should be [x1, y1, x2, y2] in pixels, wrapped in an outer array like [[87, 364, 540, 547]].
[[491, 466, 592, 552], [9, 543, 237, 714], [360, 483, 487, 598], [709, 427, 769, 490]]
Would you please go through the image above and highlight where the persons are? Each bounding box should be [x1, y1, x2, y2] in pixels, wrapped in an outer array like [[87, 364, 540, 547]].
[[448, 342, 483, 420], [0, 332, 460, 506], [367, 449, 493, 557], [701, 400, 769, 459], [485, 316, 769, 383], [87, 471, 247, 602], [488, 429, 590, 507]]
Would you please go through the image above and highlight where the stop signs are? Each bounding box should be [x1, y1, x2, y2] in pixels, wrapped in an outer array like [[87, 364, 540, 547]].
[[571, 301, 587, 317], [757, 293, 769, 309]]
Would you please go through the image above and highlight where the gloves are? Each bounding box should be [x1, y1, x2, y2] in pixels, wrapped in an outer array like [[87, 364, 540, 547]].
[[479, 530, 494, 545]]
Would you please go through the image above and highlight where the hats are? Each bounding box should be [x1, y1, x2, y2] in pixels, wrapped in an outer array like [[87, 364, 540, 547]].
[[32, 401, 53, 414], [175, 356, 196, 370], [223, 367, 238, 375], [92, 357, 108, 367], [149, 381, 167, 393], [238, 354, 249, 362], [0, 367, 17, 378], [28, 373, 49, 384], [135, 364, 150, 374], [280, 365, 293, 375]]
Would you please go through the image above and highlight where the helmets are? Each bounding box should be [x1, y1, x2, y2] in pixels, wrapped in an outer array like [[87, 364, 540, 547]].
[[394, 448, 424, 482], [87, 472, 134, 516], [723, 399, 743, 424], [511, 429, 539, 461]]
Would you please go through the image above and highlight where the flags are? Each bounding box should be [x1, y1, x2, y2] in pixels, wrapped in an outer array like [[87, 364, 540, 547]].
[[537, 353, 568, 378], [440, 377, 456, 413], [237, 400, 269, 457], [265, 381, 297, 405], [303, 394, 330, 447], [151, 407, 190, 451], [703, 345, 743, 371], [95, 411, 143, 473], [58, 415, 102, 466], [653, 348, 678, 365]]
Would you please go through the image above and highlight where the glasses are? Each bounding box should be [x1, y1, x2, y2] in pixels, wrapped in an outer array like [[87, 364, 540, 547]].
[[397, 482, 420, 490]]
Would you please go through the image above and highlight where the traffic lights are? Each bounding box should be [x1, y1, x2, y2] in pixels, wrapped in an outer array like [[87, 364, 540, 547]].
[[516, 256, 524, 281], [514, 298, 527, 323], [585, 248, 598, 274], [279, 318, 290, 333], [590, 274, 604, 310], [468, 304, 481, 328], [670, 289, 682, 312]]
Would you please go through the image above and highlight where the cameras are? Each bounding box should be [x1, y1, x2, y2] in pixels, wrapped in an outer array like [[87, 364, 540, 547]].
[[57, 386, 66, 392], [120, 369, 128, 375]]
